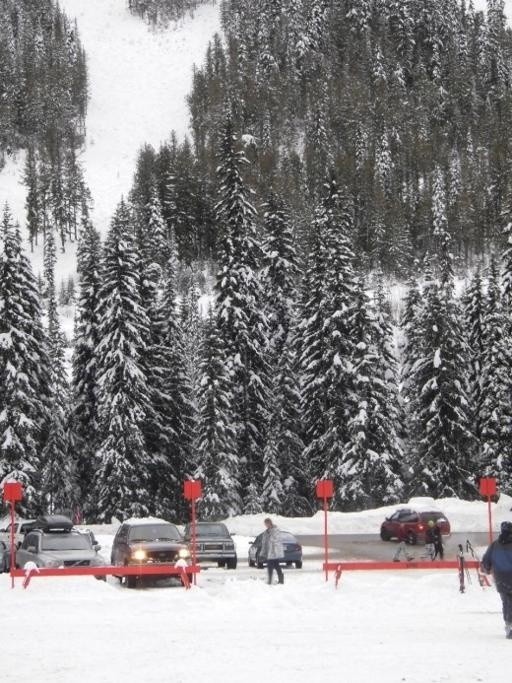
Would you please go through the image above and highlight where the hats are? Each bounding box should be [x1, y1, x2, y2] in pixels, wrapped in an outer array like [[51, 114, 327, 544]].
[[501, 522, 512, 533]]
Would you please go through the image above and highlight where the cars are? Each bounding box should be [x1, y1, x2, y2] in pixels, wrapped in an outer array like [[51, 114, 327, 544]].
[[247, 529, 304, 571]]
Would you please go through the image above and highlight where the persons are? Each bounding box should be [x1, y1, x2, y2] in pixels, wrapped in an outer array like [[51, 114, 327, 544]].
[[418, 519, 436, 561], [263, 517, 286, 585], [392, 522, 414, 563], [481, 521, 512, 640]]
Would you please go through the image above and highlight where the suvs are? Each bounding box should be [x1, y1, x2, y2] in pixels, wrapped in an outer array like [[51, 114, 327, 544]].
[[109, 520, 194, 587], [0, 516, 106, 581], [182, 521, 238, 571], [379, 507, 454, 546]]
